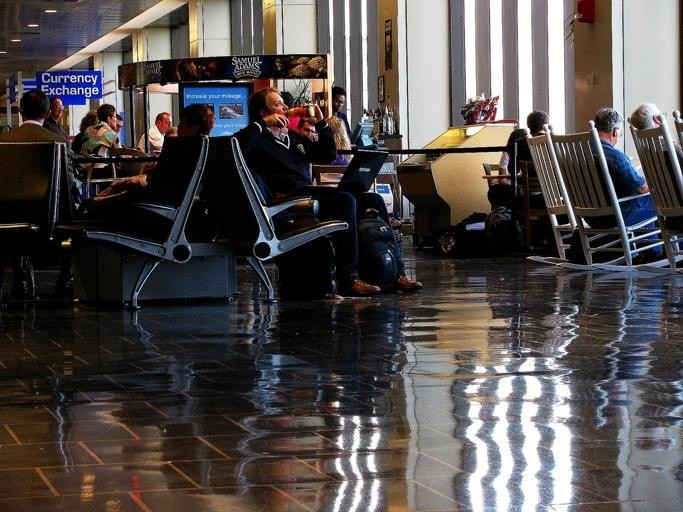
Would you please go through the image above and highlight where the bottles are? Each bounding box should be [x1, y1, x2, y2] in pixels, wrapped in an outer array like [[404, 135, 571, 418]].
[[361, 107, 396, 136], [284, 105, 316, 117]]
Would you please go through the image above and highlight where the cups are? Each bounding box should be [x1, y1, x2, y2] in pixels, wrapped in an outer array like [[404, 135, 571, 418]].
[[165, 126, 179, 137]]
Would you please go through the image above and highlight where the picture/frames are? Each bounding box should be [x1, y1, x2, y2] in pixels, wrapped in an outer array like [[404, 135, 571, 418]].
[[377, 75, 385, 102]]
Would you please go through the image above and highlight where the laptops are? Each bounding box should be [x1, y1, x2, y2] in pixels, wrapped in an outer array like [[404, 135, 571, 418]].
[[296, 149, 389, 193]]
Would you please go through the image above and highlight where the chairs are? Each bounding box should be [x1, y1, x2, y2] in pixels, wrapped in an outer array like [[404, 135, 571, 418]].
[[628, 114, 683, 271], [0, 140, 65, 303], [54, 134, 210, 310], [524, 126, 588, 262], [547, 120, 683, 269], [109, 147, 148, 182], [672, 109, 683, 143], [205, 135, 349, 304]]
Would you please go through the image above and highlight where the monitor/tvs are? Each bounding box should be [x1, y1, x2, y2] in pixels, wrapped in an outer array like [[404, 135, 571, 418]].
[[179, 82, 252, 137]]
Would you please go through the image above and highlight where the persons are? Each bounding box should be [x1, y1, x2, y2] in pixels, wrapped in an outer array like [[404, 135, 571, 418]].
[[0, 86, 423, 301], [574, 107, 666, 260], [486, 110, 563, 243], [630, 104, 683, 235]]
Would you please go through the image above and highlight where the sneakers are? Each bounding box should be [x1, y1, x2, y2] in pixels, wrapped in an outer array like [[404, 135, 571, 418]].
[[336, 278, 381, 297], [376, 271, 423, 294]]
